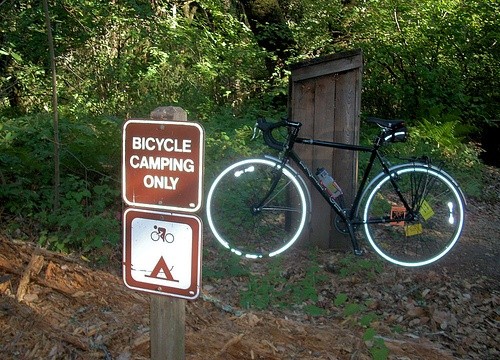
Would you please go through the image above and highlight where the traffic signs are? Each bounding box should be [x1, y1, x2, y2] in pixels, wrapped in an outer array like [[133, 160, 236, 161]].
[[120, 118, 205, 214]]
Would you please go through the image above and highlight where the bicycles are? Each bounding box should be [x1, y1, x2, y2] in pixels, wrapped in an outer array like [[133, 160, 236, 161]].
[[203, 115, 467, 268]]
